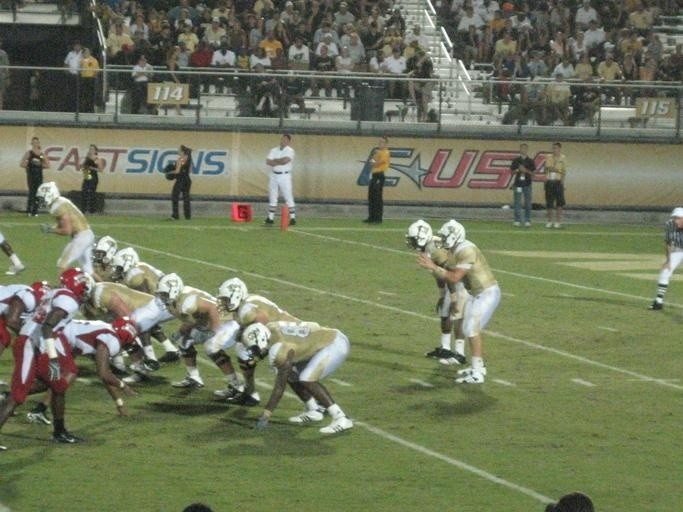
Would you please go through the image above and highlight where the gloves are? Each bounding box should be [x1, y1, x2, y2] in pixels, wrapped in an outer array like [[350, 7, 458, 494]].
[[40, 224, 50, 234]]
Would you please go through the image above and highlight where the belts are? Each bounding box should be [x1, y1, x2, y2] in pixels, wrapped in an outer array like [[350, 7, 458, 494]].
[[273, 171, 287, 174]]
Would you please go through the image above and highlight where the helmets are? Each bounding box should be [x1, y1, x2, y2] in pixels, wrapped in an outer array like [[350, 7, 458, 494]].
[[436, 220, 465, 249], [35, 182, 59, 208], [30, 233, 272, 360], [405, 220, 433, 253]]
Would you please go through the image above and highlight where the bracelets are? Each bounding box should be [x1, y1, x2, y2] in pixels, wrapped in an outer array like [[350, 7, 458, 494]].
[[431, 266, 448, 281], [438, 286, 445, 298], [516, 167, 520, 174], [262, 407, 275, 418], [450, 292, 457, 303], [113, 397, 124, 407], [44, 337, 59, 360], [119, 380, 126, 390], [557, 168, 562, 173]]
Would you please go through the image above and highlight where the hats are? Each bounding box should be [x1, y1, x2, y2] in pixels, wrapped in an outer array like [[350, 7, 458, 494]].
[[670, 207, 683, 218], [212, 17, 219, 22], [285, 1, 292, 8], [339, 2, 347, 8], [545, 491, 594, 511], [602, 41, 616, 50], [323, 33, 333, 39]]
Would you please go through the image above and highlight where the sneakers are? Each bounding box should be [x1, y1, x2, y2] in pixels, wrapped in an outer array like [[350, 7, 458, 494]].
[[25, 410, 51, 425], [426, 346, 488, 385], [647, 301, 662, 309], [263, 218, 273, 224], [289, 218, 295, 225], [319, 417, 355, 435], [6, 264, 25, 275], [1, 380, 10, 401], [290, 410, 325, 427], [513, 220, 561, 228], [116, 348, 262, 405], [51, 430, 82, 445]]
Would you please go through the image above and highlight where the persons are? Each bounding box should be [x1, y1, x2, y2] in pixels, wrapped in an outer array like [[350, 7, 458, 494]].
[[85, 1, 224, 40], [63, 42, 84, 112], [647, 207, 683, 310], [266, 134, 296, 225], [0, 279, 53, 356], [320, 0, 436, 122], [91, 234, 116, 282], [110, 247, 181, 371], [416, 219, 500, 384], [0, 49, 10, 110], [436, 0, 615, 125], [240, 321, 354, 435], [59, 267, 167, 384], [0, 267, 96, 451], [167, 144, 192, 221], [35, 181, 95, 278], [154, 272, 244, 398], [20, 137, 50, 216], [225, 1, 320, 119], [510, 143, 535, 227], [29, 70, 45, 111], [616, 0, 683, 107], [362, 136, 390, 223], [0, 315, 140, 425], [1, 234, 25, 274], [79, 144, 105, 214], [405, 219, 469, 365], [217, 277, 327, 415], [543, 143, 566, 228], [102, 40, 225, 117], [0, 0, 92, 21], [59, 268, 81, 288], [75, 49, 99, 112]]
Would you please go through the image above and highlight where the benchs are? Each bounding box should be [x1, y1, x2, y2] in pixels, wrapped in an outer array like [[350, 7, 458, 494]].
[[434, 0, 683, 130]]
